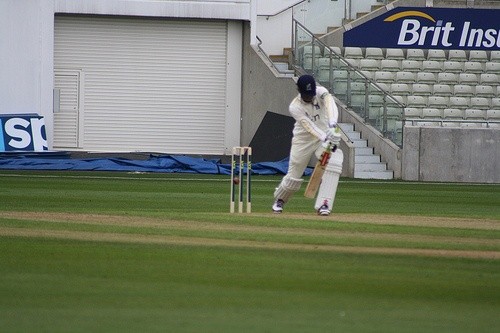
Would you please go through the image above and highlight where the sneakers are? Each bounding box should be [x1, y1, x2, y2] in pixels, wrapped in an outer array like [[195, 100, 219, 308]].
[[319, 204, 330, 215], [272, 199, 284, 211]]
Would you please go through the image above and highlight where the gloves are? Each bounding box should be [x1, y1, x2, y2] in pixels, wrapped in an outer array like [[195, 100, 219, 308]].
[[323, 127, 341, 149]]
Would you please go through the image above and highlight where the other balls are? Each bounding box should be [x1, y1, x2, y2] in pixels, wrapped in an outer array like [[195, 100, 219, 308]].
[[234, 177, 239, 184]]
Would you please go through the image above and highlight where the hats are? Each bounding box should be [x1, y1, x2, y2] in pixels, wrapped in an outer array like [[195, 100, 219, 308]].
[[297, 75, 316, 96]]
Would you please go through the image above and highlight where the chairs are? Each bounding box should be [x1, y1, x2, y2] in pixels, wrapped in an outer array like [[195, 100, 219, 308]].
[[301, 48, 500, 145]]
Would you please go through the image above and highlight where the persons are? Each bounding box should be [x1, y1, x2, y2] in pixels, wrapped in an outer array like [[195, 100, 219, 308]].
[[272, 74, 344, 215]]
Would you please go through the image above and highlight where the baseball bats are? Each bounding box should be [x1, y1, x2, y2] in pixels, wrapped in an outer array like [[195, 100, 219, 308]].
[[304, 126, 340, 199]]
